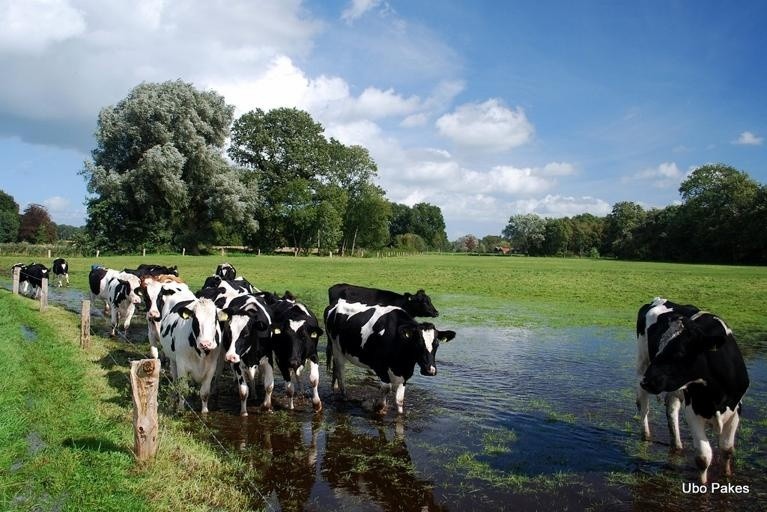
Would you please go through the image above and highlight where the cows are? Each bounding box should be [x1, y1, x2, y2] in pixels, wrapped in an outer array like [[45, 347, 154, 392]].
[[52, 258, 69, 288], [634, 296, 750, 485], [89, 261, 324, 418], [11, 262, 51, 299], [323, 297, 456, 415], [328, 282, 439, 321]]
